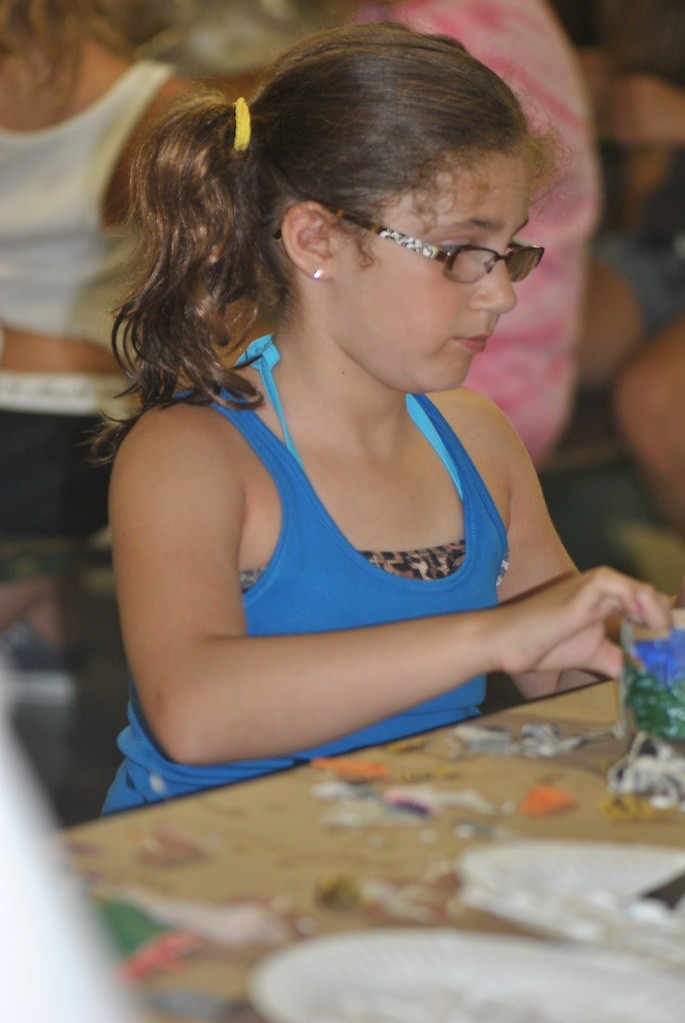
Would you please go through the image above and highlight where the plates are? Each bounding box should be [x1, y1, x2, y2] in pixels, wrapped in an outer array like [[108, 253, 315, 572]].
[[250, 927, 685, 1023], [457, 844, 685, 962]]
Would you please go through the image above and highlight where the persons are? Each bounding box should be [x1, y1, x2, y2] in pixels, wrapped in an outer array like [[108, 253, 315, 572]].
[[292, 1, 598, 472], [101, 21, 685, 819], [551, 0, 683, 541], [0, 0, 201, 541]]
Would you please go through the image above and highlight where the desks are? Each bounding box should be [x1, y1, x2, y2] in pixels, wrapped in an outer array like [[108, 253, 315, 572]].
[[62, 680, 685, 1023]]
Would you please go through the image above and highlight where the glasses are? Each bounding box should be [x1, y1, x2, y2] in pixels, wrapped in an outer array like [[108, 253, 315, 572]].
[[272, 195, 547, 283]]
